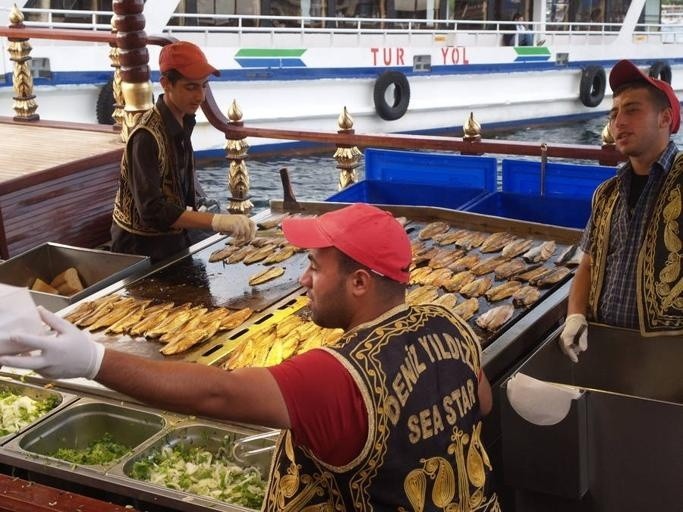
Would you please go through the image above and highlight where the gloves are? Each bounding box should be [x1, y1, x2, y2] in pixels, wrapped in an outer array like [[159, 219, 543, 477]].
[[0, 306, 105, 381], [211, 211, 256, 240], [560, 312, 588, 362]]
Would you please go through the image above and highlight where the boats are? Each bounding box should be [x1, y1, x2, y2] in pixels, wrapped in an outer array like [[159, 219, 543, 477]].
[[0, 0, 683, 162]]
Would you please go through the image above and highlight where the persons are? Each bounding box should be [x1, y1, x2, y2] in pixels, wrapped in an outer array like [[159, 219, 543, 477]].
[[110, 41, 256, 265], [504, 14, 533, 46], [0, 203, 506, 512], [558, 78, 683, 364]]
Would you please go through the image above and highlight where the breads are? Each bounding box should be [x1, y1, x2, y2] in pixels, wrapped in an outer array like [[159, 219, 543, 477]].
[[32, 266, 83, 296]]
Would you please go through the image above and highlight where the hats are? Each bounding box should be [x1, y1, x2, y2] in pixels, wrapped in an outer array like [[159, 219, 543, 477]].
[[282, 203, 412, 285], [610, 60, 682, 133], [159, 42, 220, 80]]
